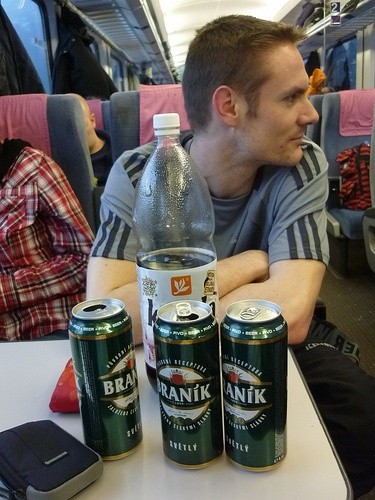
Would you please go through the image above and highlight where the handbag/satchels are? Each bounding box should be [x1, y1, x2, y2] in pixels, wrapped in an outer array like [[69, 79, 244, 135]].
[[0, 419, 104, 500]]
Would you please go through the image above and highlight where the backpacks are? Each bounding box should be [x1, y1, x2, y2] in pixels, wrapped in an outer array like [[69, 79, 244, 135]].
[[335, 143, 370, 211]]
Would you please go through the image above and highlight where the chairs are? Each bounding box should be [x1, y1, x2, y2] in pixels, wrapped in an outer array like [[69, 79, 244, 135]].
[[0, 85, 375, 320]]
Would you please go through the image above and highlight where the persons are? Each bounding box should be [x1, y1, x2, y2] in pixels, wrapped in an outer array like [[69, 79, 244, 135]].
[[75, 95, 112, 186], [0, 138, 96, 341], [85, 14, 375, 500]]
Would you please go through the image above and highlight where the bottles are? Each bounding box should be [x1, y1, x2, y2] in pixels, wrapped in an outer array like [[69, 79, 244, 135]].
[[131, 112, 220, 394]]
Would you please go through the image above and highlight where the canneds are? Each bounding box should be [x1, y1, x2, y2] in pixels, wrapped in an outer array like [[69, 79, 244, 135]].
[[68, 299, 143, 461], [153, 300, 224, 469], [220, 299, 288, 472]]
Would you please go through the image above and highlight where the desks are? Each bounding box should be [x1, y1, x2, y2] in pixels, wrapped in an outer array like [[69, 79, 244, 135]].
[[0, 339, 355, 500]]
